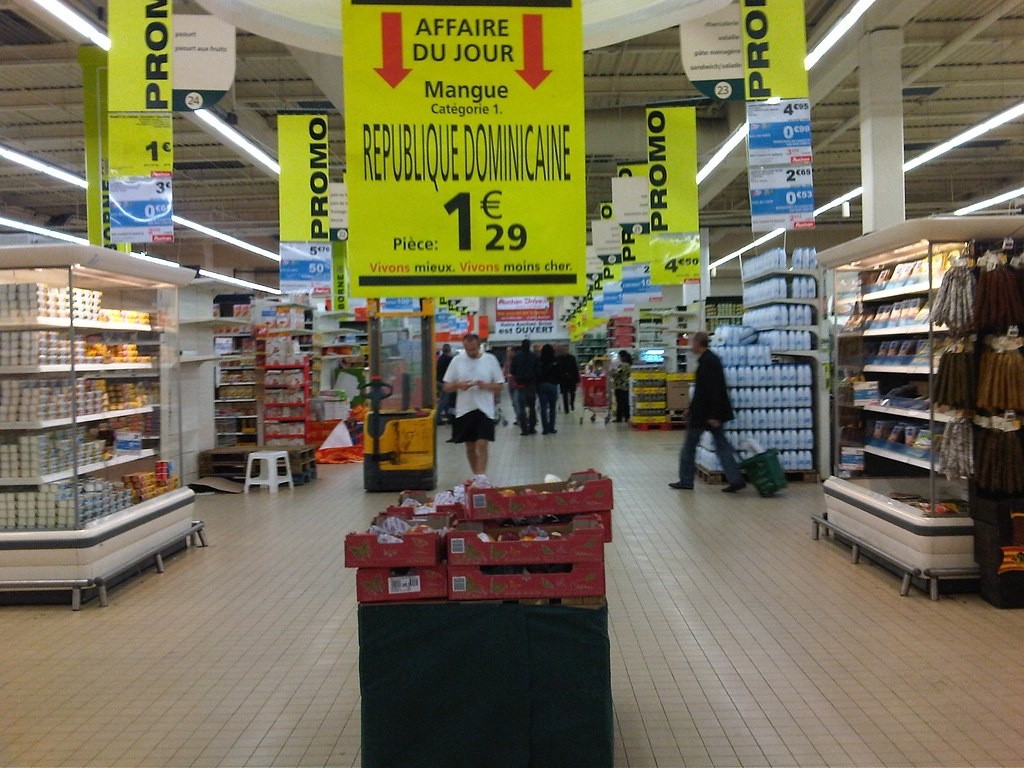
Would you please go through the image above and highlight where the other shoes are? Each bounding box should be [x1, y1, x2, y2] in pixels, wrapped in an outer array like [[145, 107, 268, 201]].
[[612, 418, 622, 423], [722, 479, 747, 492], [549, 428, 557, 433], [529, 430, 537, 434], [669, 482, 693, 489], [520, 432, 529, 436], [542, 429, 549, 434]]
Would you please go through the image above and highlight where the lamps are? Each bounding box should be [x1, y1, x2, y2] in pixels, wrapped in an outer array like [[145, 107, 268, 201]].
[[842, 200, 850, 218]]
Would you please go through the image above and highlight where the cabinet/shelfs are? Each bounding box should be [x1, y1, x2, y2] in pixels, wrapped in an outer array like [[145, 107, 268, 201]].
[[742, 267, 831, 480], [0, 316, 193, 605], [576, 300, 743, 431], [107, 277, 370, 488]]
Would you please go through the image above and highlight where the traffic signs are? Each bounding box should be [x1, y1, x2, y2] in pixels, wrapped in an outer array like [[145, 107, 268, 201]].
[[344, 1, 588, 298]]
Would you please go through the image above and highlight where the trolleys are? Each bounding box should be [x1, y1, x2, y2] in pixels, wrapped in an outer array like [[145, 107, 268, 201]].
[[360, 296, 437, 492], [579, 373, 613, 425]]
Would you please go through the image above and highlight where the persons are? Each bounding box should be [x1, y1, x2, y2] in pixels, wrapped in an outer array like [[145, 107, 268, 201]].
[[503, 339, 581, 436], [436, 344, 454, 424], [669, 332, 746, 492], [606, 350, 633, 422], [442, 335, 506, 475]]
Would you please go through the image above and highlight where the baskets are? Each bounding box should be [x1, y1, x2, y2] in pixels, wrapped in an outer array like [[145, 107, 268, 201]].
[[738, 448, 787, 496]]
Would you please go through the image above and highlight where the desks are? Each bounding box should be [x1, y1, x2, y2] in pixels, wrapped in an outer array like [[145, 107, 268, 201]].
[[358, 598, 616, 768]]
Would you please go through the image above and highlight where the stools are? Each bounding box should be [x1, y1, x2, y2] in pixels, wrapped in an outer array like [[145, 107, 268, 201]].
[[244, 450, 293, 495]]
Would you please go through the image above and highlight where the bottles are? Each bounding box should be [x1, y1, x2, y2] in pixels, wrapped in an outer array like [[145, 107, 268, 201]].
[[742, 247, 817, 325], [688, 323, 817, 471]]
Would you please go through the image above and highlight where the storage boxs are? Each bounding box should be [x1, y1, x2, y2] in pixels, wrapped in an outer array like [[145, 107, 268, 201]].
[[345, 469, 614, 602], [667, 382, 691, 409]]
